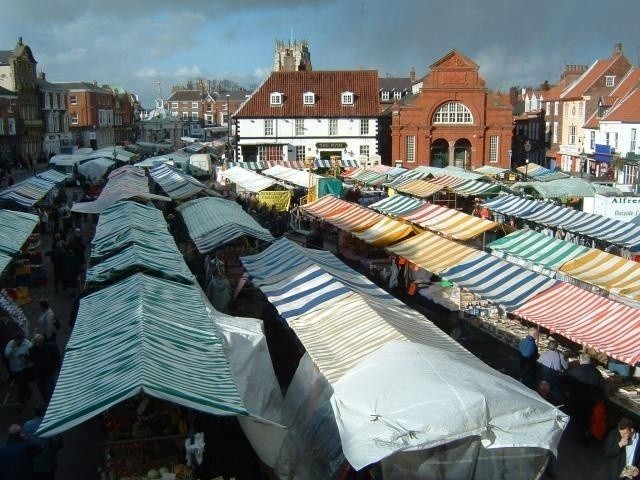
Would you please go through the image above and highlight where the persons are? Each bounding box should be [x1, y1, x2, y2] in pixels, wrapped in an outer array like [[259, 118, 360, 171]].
[[153, 183, 177, 234], [604, 417, 640, 480], [211, 185, 323, 250], [519, 326, 605, 446], [1, 151, 105, 480]]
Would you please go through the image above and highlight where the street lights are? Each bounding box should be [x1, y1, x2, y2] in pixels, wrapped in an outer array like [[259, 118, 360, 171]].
[[525, 154, 529, 182]]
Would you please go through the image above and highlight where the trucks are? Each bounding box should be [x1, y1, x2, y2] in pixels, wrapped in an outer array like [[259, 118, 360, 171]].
[[189, 154, 213, 180]]
